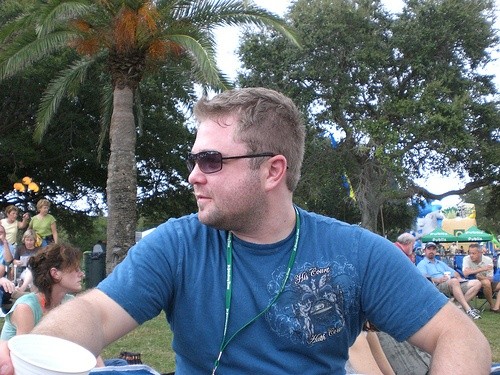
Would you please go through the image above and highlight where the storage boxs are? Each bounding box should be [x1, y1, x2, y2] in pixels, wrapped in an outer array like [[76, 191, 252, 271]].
[[89, 365, 160, 375]]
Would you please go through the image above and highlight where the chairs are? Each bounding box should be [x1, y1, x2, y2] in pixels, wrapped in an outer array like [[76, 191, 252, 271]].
[[415, 254, 449, 298], [454, 255, 497, 309]]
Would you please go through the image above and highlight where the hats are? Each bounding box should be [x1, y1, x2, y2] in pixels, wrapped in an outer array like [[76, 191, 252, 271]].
[[425, 242, 437, 249]]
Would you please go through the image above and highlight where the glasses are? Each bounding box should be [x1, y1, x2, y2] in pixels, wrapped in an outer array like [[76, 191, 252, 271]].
[[186, 150, 274, 173]]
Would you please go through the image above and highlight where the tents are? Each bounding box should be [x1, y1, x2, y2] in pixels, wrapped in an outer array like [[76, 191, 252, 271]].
[[420, 225, 493, 255]]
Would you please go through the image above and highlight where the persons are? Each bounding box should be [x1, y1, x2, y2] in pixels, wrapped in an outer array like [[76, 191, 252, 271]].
[[392, 232, 500, 320], [91, 240, 104, 254], [1, 240, 85, 341], [0, 86, 492, 375], [0, 199, 59, 320]]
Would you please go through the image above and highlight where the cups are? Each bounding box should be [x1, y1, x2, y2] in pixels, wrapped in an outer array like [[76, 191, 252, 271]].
[[488, 264, 493, 272], [7, 334, 97, 375], [444, 271, 451, 281]]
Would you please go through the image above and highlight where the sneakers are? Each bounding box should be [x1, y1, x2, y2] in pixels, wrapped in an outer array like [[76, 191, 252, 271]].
[[467, 308, 481, 320]]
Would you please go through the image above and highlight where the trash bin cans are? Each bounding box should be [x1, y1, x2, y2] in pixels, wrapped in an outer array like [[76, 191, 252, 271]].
[[84, 253, 106, 289]]
